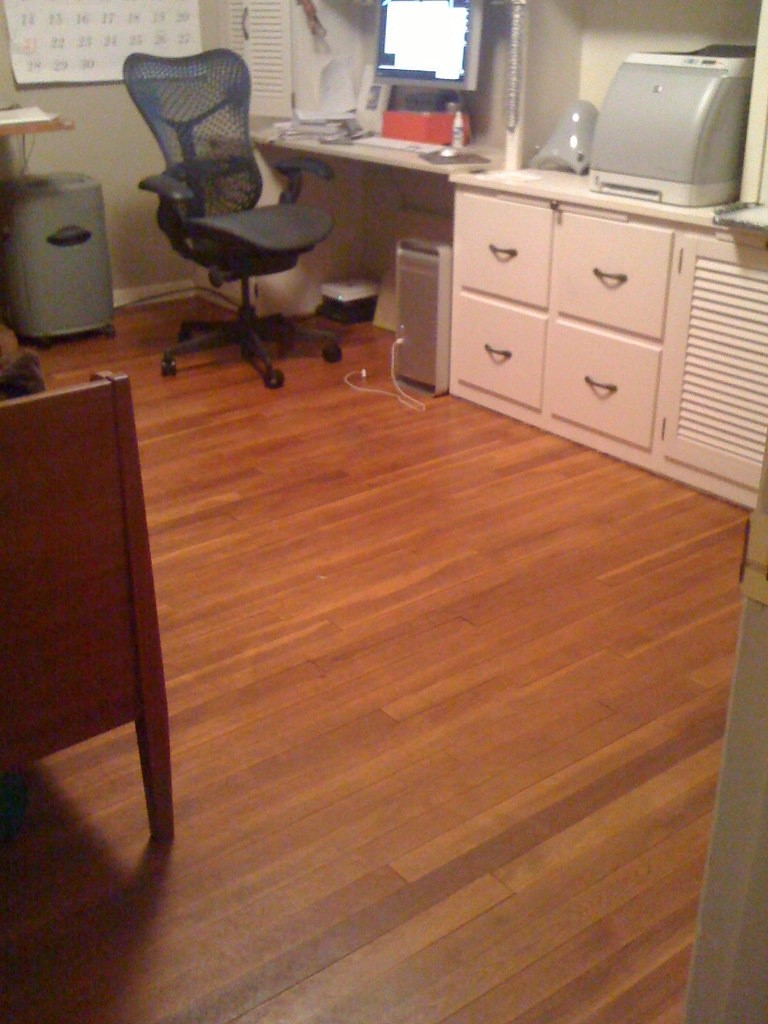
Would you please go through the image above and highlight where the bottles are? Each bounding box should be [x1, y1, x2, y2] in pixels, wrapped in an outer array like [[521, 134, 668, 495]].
[[453, 111, 463, 149]]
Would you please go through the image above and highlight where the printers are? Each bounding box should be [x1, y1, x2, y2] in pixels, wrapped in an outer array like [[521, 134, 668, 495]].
[[589, 44, 755, 209]]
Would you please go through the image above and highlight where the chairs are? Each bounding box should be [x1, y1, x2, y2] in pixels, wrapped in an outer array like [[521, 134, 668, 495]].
[[123, 48, 342, 389]]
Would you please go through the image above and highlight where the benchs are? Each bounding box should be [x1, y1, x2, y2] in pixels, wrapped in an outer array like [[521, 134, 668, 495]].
[[0, 371, 175, 843]]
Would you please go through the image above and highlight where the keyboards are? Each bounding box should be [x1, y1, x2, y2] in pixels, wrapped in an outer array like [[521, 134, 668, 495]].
[[353, 136, 447, 154]]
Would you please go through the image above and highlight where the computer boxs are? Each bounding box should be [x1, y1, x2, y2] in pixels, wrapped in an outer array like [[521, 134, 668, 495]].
[[395, 238, 453, 397]]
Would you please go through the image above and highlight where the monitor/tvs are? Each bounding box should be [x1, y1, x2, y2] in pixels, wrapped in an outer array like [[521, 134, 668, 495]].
[[374, 0, 484, 115]]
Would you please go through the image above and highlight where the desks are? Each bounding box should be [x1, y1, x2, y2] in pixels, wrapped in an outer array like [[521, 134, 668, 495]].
[[0, 119, 74, 135], [249, 123, 505, 318]]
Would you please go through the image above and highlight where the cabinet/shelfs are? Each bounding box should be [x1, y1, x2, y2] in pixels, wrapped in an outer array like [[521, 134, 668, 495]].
[[195, 0, 362, 324], [448, 0, 768, 513]]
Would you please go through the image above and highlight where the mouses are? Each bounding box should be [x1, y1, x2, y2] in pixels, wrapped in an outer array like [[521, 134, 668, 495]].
[[439, 147, 463, 158]]
